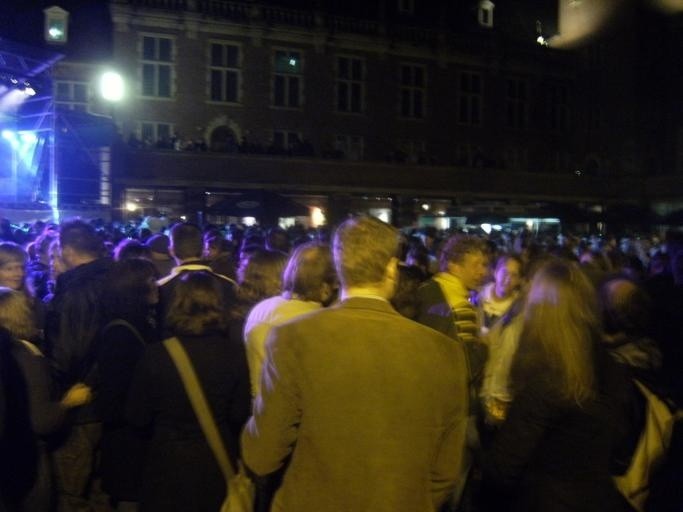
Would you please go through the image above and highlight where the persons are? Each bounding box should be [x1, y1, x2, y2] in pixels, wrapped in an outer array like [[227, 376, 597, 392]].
[[596, 277, 661, 384], [239, 213, 466, 512], [0, 217, 57, 247], [96, 259, 160, 512], [411, 234, 489, 436], [204, 218, 338, 256], [41, 239, 68, 302], [469, 258, 648, 512], [244, 242, 340, 512], [130, 268, 253, 512], [0, 287, 93, 511], [1, 243, 44, 344], [46, 222, 110, 512], [100, 211, 170, 260], [26, 232, 57, 302], [393, 222, 485, 270], [234, 252, 284, 352], [154, 222, 236, 317], [477, 256, 524, 335], [126, 129, 343, 158], [486, 226, 681, 271]]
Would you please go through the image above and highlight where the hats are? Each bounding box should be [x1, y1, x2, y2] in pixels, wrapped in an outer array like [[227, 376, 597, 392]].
[[141, 235, 171, 252], [414, 224, 441, 241], [240, 234, 267, 252]]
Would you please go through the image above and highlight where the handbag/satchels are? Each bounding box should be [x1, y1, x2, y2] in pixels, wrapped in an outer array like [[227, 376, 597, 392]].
[[604, 373, 682, 511], [52, 427, 110, 512]]
